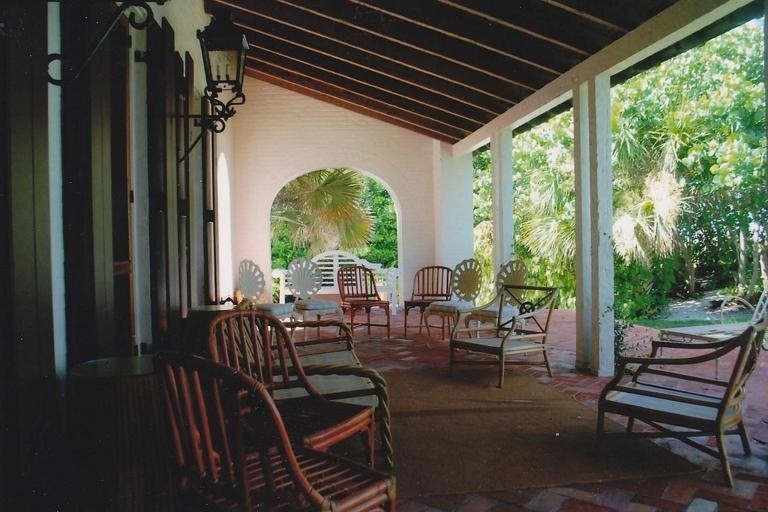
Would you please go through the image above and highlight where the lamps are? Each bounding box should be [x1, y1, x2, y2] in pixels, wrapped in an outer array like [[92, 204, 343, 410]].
[[167, 5, 252, 163]]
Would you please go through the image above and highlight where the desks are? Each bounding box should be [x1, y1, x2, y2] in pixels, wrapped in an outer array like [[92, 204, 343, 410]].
[[73, 353, 163, 511]]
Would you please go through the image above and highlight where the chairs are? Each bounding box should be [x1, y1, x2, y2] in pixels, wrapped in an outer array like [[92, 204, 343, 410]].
[[209, 312, 377, 468], [164, 339, 395, 511], [464, 259, 527, 353], [597, 319, 768, 486], [424, 259, 482, 349], [287, 259, 343, 341], [448, 284, 559, 388], [337, 264, 390, 340], [224, 301, 394, 470], [239, 260, 294, 345], [405, 265, 454, 342]]
